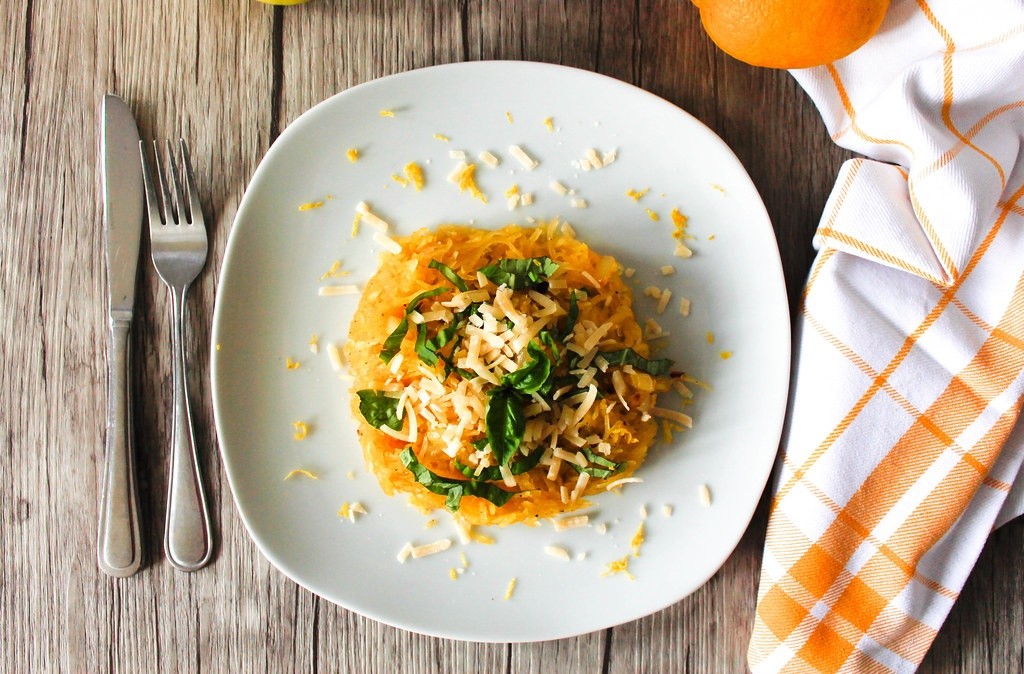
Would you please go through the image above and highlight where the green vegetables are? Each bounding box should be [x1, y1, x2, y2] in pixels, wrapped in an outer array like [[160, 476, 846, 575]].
[[355, 256, 684, 511]]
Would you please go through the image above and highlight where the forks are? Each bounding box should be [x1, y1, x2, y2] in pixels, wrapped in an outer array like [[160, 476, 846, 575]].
[[138, 139, 211, 569]]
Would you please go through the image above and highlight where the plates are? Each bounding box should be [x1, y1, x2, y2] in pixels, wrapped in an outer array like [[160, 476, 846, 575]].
[[210, 58, 792, 643]]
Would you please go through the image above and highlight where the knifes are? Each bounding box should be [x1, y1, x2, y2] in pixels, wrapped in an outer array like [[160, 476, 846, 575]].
[[96, 95, 145, 578]]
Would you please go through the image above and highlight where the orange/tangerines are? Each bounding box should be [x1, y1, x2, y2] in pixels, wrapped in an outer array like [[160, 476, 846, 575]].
[[692, 0, 890, 69]]
